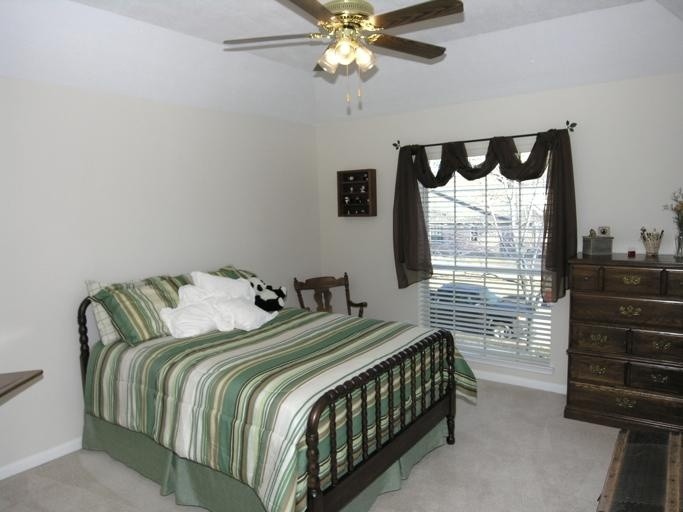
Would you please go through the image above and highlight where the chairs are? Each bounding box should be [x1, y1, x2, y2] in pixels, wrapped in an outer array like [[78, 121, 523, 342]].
[[293, 272, 367, 317]]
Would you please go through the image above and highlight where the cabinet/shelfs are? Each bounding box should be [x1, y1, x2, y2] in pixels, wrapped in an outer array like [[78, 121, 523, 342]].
[[563, 254, 683, 435], [337, 169, 377, 217]]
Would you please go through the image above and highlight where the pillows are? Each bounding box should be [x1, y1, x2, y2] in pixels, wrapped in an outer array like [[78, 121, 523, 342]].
[[82, 265, 279, 348]]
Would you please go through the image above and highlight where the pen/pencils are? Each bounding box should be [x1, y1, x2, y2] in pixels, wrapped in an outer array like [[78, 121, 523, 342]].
[[640, 226, 664, 241]]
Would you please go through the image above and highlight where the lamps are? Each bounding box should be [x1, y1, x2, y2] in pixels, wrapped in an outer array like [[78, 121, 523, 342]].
[[317, 33, 376, 104]]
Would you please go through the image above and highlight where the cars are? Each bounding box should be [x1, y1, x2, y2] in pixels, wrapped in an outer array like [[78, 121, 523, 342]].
[[430, 282, 550, 340]]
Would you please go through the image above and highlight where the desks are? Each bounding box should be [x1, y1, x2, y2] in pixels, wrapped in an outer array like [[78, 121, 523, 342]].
[[0, 369, 44, 408]]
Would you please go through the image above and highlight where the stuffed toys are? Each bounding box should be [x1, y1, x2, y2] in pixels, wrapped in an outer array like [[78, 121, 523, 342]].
[[246, 274, 287, 312]]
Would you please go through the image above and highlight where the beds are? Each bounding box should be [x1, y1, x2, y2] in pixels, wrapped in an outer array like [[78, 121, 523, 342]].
[[78, 297, 478, 512]]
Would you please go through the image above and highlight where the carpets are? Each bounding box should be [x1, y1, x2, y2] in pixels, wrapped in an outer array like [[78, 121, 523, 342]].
[[595, 428, 683, 512]]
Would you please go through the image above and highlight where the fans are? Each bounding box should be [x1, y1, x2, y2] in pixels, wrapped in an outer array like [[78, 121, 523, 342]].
[[223, 0, 463, 59]]
